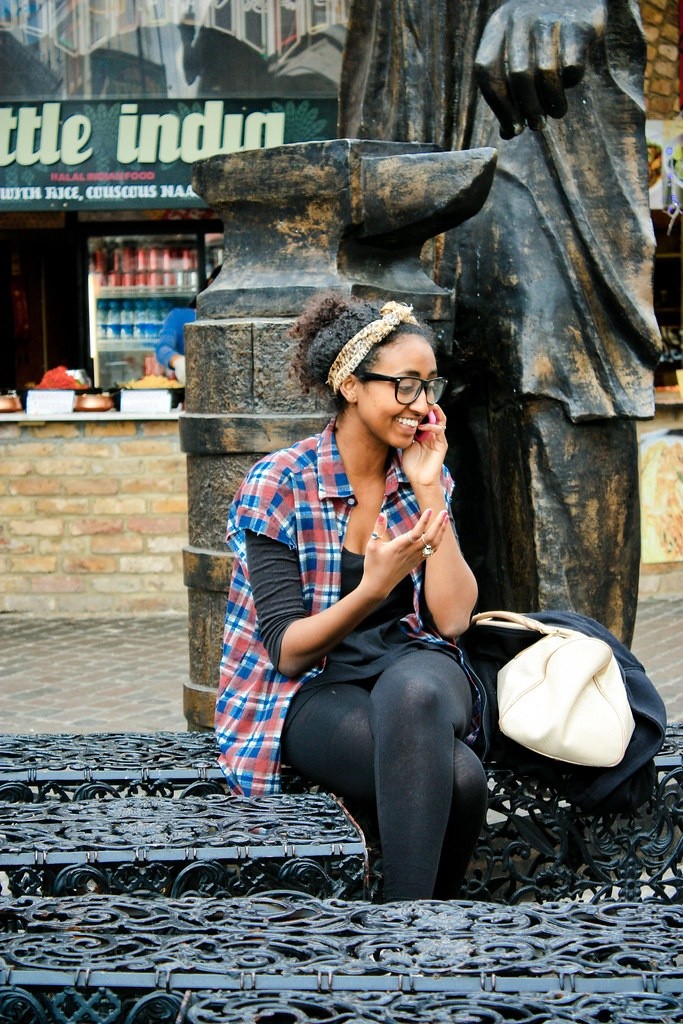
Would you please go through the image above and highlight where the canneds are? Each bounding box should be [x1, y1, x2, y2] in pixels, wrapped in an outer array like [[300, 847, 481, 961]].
[[95, 244, 200, 291]]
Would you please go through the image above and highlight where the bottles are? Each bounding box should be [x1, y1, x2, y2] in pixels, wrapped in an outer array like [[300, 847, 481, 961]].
[[95, 299, 176, 340]]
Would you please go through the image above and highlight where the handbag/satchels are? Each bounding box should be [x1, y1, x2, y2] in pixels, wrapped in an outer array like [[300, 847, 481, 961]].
[[472, 610, 635, 767]]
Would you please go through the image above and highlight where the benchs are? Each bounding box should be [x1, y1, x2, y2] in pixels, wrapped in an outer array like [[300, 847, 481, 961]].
[[0, 896, 683, 1024], [0, 792, 370, 902], [0, 722, 683, 905]]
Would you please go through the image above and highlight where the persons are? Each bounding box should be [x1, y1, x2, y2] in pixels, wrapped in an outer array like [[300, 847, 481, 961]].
[[213, 288, 488, 902], [153, 263, 223, 386]]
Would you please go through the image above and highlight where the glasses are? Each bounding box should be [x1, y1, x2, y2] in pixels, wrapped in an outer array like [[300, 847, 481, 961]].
[[352, 370, 448, 405]]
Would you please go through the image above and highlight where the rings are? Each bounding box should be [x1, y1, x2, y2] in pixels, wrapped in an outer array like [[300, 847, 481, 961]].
[[422, 546, 434, 558], [407, 531, 418, 543], [370, 532, 382, 540], [421, 533, 432, 550]]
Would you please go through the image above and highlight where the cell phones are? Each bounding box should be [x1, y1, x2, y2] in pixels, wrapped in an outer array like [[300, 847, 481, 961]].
[[414, 411, 437, 443]]
